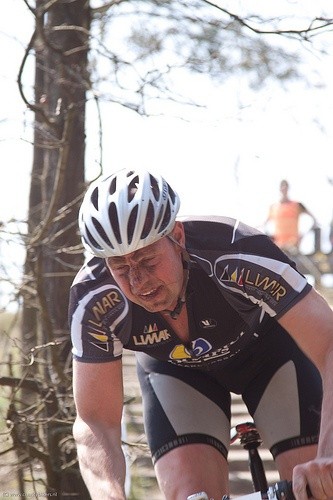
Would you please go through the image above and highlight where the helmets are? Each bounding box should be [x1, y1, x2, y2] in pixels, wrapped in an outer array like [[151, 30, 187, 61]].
[[78, 167, 181, 258]]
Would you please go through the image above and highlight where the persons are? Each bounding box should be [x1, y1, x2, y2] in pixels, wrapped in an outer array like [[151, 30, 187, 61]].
[[70, 166, 333, 500], [265, 178, 318, 263]]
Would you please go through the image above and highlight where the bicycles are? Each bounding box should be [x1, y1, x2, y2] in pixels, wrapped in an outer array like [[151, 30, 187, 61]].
[[186, 417, 315, 500]]
